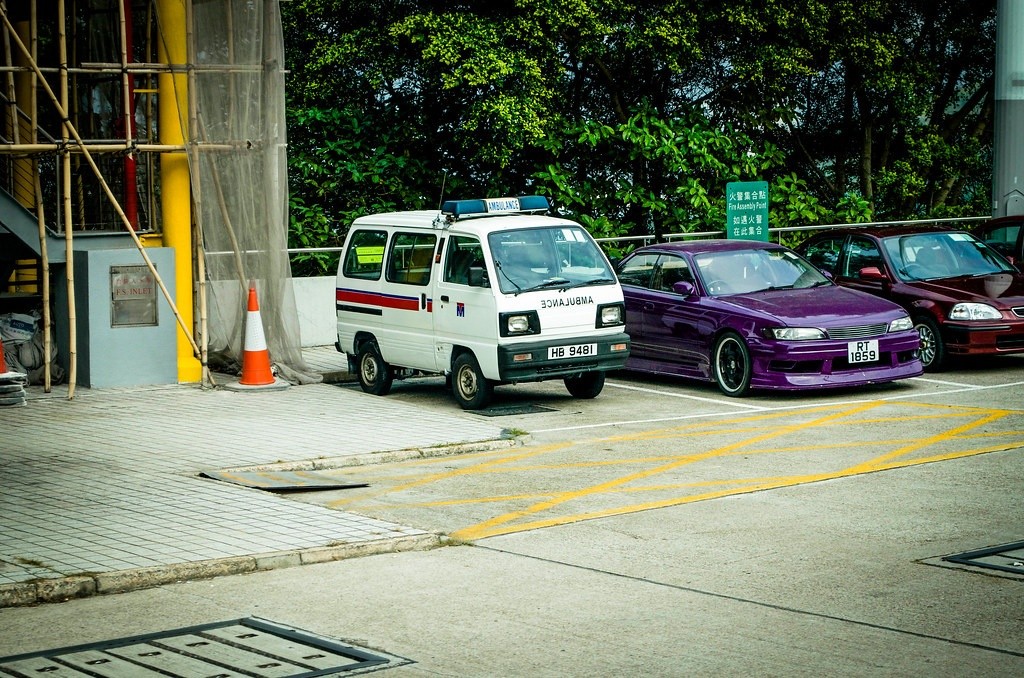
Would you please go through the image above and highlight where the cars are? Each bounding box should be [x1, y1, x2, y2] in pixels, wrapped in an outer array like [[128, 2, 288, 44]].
[[974, 214, 1024, 276], [794, 223, 1024, 372], [610, 238, 923, 397]]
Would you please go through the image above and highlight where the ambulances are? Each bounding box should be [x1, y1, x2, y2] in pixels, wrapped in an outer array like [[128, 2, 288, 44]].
[[332, 193, 633, 411]]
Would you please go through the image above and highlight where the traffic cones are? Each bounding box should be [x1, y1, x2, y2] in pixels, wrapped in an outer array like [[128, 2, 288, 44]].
[[0, 340, 27, 407], [237, 288, 278, 387]]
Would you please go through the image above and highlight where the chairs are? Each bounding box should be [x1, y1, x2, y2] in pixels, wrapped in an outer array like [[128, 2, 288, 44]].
[[915, 248, 951, 276], [450, 249, 475, 285], [859, 246, 882, 278]]
